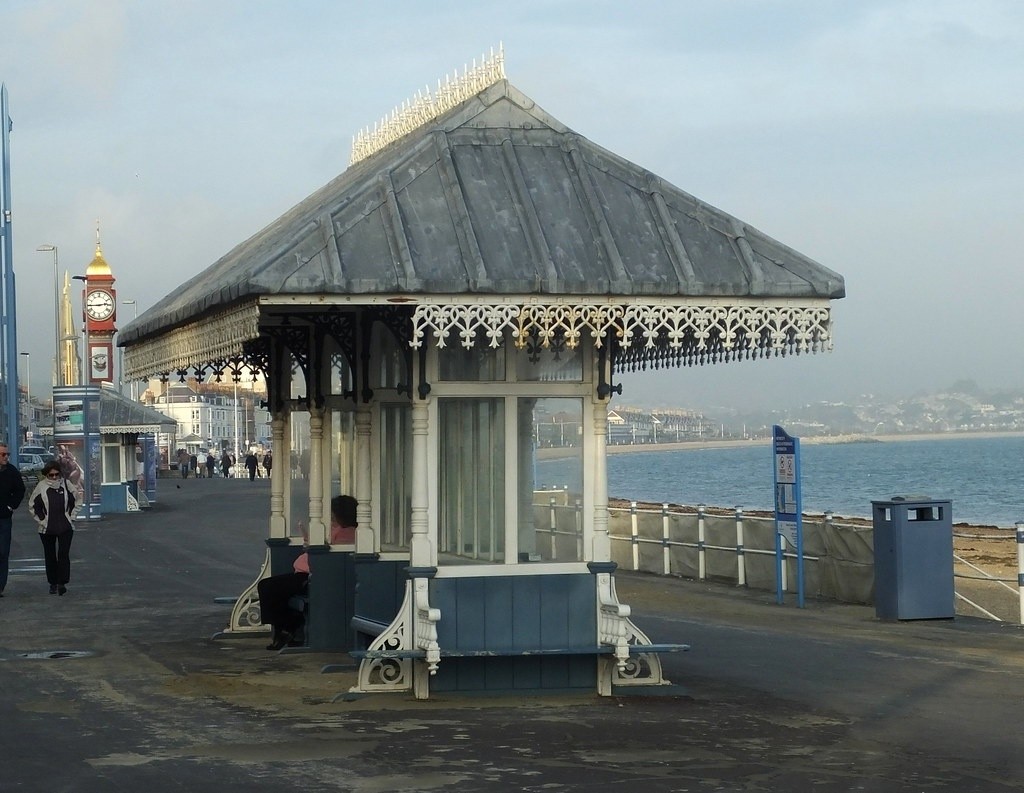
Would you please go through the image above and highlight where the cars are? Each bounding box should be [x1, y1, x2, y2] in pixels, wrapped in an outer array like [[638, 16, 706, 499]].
[[9, 438, 54, 476]]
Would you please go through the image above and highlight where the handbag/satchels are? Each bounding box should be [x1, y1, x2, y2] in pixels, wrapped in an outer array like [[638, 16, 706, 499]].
[[257, 467, 261, 478], [65, 478, 75, 514]]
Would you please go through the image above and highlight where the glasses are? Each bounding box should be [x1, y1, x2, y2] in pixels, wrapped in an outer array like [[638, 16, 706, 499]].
[[48, 473, 60, 477], [0, 452, 10, 457]]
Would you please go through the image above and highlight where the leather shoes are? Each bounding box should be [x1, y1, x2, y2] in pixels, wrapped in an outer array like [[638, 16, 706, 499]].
[[288, 638, 304, 647], [267, 629, 293, 650]]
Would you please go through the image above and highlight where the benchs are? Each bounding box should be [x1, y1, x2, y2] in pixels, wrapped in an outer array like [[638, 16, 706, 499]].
[[101, 480, 151, 513], [224, 538, 690, 700], [21, 472, 40, 498]]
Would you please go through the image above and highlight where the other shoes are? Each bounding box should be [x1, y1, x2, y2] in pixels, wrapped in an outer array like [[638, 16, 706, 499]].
[[49, 586, 57, 594], [58, 586, 66, 595]]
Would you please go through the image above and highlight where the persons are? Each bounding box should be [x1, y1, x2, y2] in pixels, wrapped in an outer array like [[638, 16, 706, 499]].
[[177, 447, 231, 478], [0, 442, 26, 598], [290, 449, 310, 480], [244, 451, 258, 481], [257, 495, 359, 651], [262, 451, 273, 479], [28, 462, 75, 596]]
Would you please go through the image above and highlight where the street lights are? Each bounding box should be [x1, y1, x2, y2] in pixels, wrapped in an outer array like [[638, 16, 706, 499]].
[[72, 275, 88, 385], [35, 244, 62, 387], [123, 299, 140, 403], [20, 352, 30, 431]]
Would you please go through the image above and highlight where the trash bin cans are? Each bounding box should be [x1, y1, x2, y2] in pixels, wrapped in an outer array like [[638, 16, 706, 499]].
[[870, 498, 957, 622]]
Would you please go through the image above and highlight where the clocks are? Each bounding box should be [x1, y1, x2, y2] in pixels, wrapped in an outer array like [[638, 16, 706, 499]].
[[83, 289, 115, 322]]
